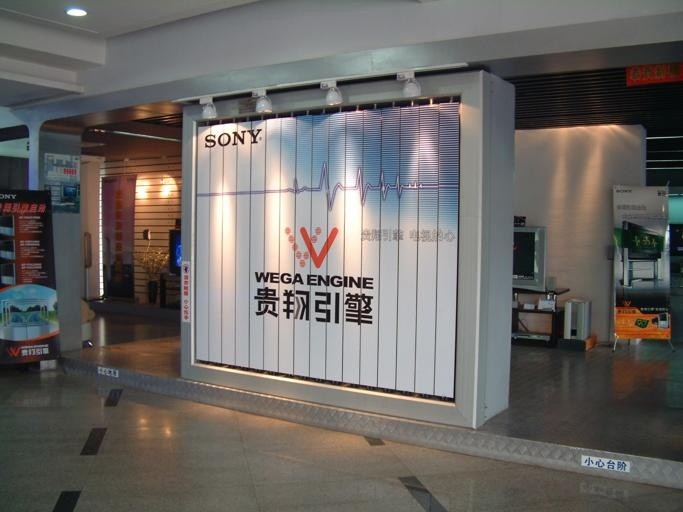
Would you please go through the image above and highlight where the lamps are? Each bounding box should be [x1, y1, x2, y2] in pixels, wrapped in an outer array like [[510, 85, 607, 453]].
[[320, 81, 344, 106], [199, 96, 217, 119], [251, 90, 273, 113], [397, 71, 423, 98]]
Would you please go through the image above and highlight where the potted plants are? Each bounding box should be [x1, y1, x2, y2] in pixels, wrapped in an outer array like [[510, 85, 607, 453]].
[[131, 248, 170, 305]]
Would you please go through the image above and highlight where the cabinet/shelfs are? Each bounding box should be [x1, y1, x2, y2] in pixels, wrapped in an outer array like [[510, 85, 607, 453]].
[[512, 287, 569, 348]]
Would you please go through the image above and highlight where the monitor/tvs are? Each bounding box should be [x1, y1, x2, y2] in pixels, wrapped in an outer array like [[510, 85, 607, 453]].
[[168, 228, 181, 276], [514, 225, 548, 292]]
